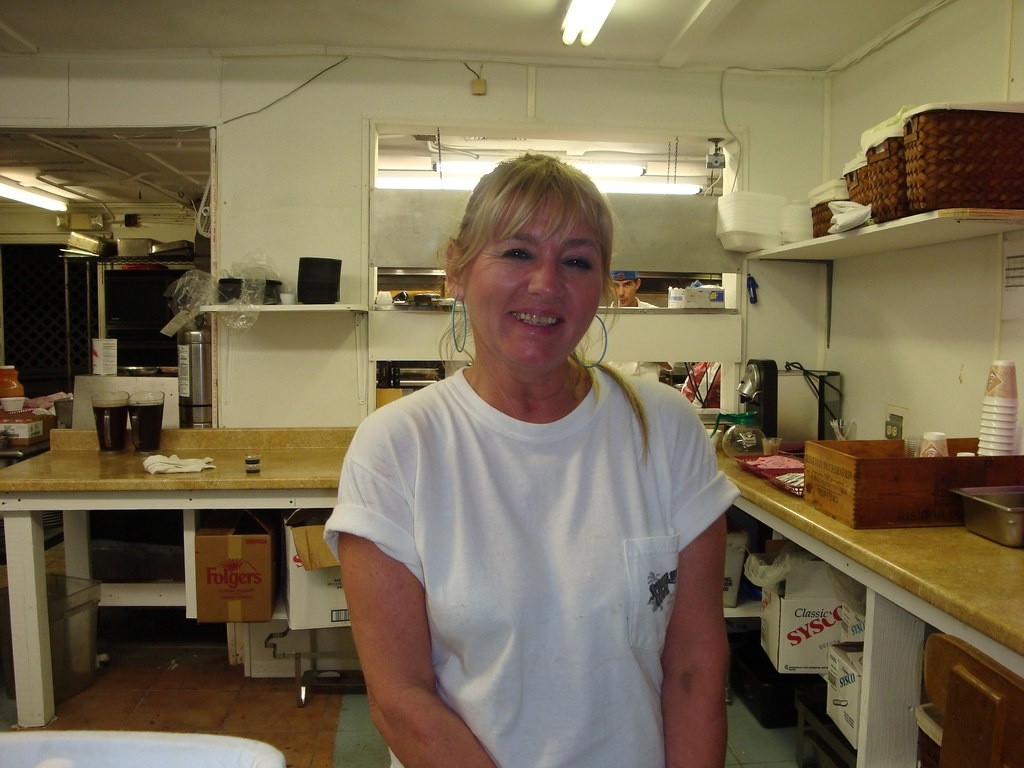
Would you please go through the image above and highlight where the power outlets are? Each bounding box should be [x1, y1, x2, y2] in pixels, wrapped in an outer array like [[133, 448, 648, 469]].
[[885, 421, 902, 440]]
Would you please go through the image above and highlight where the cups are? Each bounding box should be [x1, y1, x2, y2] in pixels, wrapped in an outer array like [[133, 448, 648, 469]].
[[413, 294, 431, 305], [91, 390, 130, 456], [977, 360, 1018, 456], [376, 292, 393, 304], [705, 429, 722, 452], [956, 452, 974, 455], [245, 454, 261, 473], [920, 432, 949, 457], [128, 391, 165, 455], [761, 437, 783, 455]]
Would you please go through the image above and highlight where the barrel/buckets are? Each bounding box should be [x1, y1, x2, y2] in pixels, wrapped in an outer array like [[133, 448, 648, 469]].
[[49, 396, 74, 429]]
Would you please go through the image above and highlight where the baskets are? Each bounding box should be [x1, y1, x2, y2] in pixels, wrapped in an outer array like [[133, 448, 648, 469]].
[[902, 101, 1024, 210], [806, 105, 921, 238]]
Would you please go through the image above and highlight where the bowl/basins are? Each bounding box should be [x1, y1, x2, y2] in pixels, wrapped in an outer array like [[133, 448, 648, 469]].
[[0, 397, 26, 411], [716, 191, 787, 252], [280, 294, 296, 305]]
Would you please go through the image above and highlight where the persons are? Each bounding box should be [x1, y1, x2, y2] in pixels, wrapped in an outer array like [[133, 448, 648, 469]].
[[607, 269, 676, 382], [325, 153, 743, 768]]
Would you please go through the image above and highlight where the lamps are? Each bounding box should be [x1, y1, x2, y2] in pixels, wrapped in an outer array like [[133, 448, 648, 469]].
[[374, 162, 703, 195], [558, 0, 617, 46], [56, 213, 104, 231], [0, 175, 70, 212]]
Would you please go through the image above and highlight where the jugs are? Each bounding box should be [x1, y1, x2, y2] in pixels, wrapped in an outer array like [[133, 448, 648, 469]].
[[710, 411, 766, 460]]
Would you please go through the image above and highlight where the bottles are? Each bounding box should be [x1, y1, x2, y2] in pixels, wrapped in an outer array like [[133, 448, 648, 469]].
[[0, 366, 24, 397], [784, 199, 813, 243]]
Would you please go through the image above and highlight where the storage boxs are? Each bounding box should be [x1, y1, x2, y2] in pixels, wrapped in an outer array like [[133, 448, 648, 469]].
[[826, 645, 864, 749], [668, 287, 725, 308], [0, 573, 101, 705], [195, 510, 273, 621], [840, 602, 866, 643], [723, 514, 748, 608], [284, 509, 351, 629], [0, 417, 55, 445], [804, 438, 1023, 530], [749, 539, 867, 673]]
